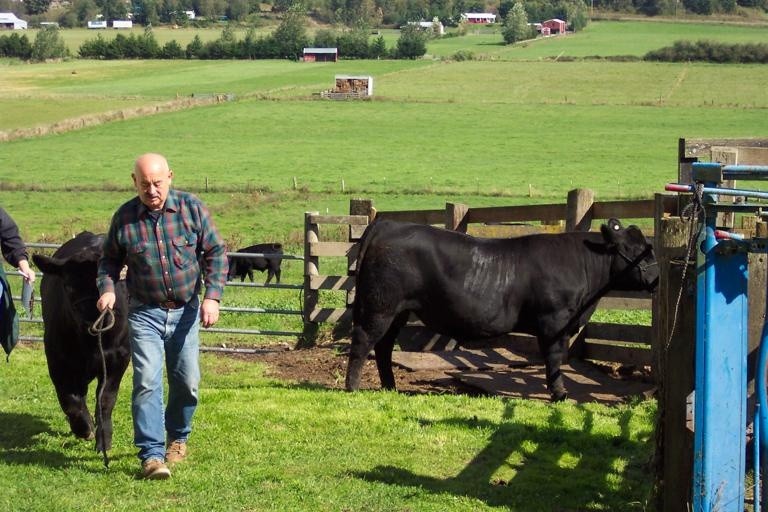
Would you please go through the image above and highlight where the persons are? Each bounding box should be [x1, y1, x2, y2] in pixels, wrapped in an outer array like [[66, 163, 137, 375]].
[[95, 153, 230, 481], [0, 207, 36, 285]]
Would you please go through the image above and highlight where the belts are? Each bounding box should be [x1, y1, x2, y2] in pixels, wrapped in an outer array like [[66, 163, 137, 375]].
[[154, 300, 183, 310]]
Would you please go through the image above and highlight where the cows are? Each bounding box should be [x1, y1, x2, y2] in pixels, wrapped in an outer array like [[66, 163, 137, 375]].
[[344, 216, 661, 404], [227, 242, 284, 288], [30, 229, 132, 454]]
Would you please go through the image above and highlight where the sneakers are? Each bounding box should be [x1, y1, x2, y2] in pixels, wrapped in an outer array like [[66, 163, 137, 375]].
[[143, 458, 169, 480], [164, 441, 187, 463]]
